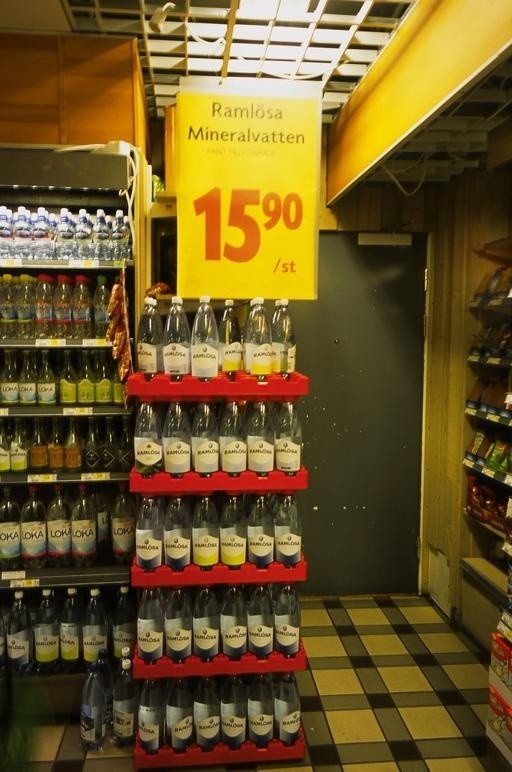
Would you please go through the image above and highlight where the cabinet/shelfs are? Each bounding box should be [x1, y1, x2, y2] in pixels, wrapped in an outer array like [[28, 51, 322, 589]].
[[461, 235, 512, 661], [1, 150, 150, 719], [126, 296, 311, 769]]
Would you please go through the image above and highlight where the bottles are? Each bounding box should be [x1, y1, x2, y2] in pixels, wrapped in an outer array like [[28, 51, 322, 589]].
[[95, 648, 114, 728], [53, 274, 71, 337], [34, 273, 53, 338], [32, 206, 54, 259], [0, 349, 18, 404], [77, 349, 95, 405], [113, 360, 125, 406], [0, 418, 10, 473], [219, 396, 248, 478], [0, 273, 16, 338], [136, 493, 164, 573], [47, 416, 64, 473], [92, 209, 111, 260], [162, 396, 191, 479], [118, 415, 135, 471], [93, 275, 111, 339], [79, 665, 107, 750], [248, 582, 275, 661], [247, 673, 273, 750], [242, 299, 254, 375], [275, 489, 303, 568], [191, 396, 219, 478], [6, 590, 34, 666], [13, 206, 33, 259], [133, 395, 164, 478], [272, 298, 297, 381], [74, 208, 92, 259], [101, 415, 118, 471], [220, 490, 248, 571], [64, 416, 82, 473], [113, 646, 132, 682], [250, 296, 273, 382], [193, 584, 220, 663], [37, 348, 57, 405], [166, 677, 194, 754], [246, 396, 275, 478], [162, 295, 191, 382], [136, 297, 163, 382], [29, 417, 47, 472], [47, 483, 71, 568], [21, 485, 47, 569], [138, 678, 166, 755], [95, 349, 113, 405], [91, 481, 110, 546], [112, 658, 138, 748], [16, 274, 34, 338], [274, 395, 303, 476], [275, 582, 302, 659], [60, 587, 83, 663], [192, 490, 220, 571], [273, 671, 301, 746], [220, 583, 248, 661], [110, 481, 136, 562], [165, 585, 194, 664], [71, 275, 92, 339], [55, 207, 74, 260], [71, 483, 98, 568], [0, 205, 13, 259], [59, 350, 77, 405], [193, 676, 221, 753], [83, 587, 109, 663], [10, 417, 28, 472], [137, 586, 165, 665], [34, 588, 60, 664], [248, 490, 274, 569], [218, 299, 242, 381], [18, 349, 37, 404], [221, 674, 247, 750], [0, 485, 21, 571], [82, 416, 102, 472], [164, 492, 192, 573], [191, 295, 218, 382], [112, 586, 137, 660], [111, 209, 131, 260]]
